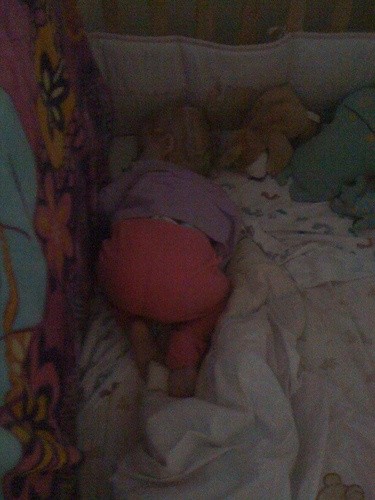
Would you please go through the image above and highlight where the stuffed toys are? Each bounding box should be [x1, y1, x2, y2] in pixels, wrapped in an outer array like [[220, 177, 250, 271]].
[[271, 86, 375, 239], [214, 81, 323, 183]]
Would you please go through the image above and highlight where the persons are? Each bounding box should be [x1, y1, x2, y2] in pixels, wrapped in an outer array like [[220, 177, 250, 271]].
[[94, 103, 244, 400]]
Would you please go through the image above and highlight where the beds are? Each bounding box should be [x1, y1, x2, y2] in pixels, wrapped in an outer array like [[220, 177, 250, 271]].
[[72, 132, 375, 500]]
